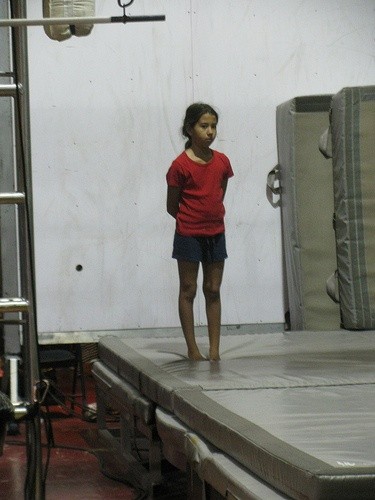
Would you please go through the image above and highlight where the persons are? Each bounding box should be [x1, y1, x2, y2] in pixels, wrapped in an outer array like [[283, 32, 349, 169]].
[[0, 390, 16, 458], [165, 103, 234, 362]]
